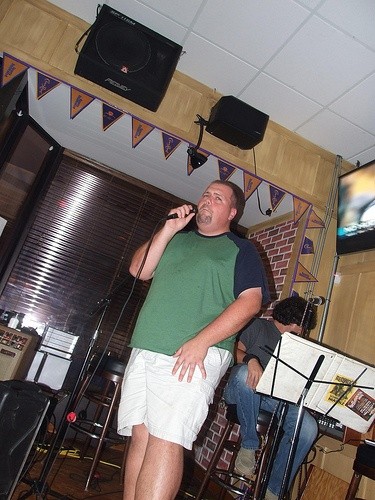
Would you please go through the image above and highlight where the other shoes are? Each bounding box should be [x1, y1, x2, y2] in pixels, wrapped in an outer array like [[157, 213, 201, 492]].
[[235, 447, 255, 476], [265, 489, 279, 500]]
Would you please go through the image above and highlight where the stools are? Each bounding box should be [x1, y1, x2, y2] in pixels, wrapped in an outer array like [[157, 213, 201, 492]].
[[193, 400, 284, 500], [39, 349, 131, 491]]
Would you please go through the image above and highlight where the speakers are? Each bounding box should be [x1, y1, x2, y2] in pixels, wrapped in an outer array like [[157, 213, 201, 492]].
[[0, 379, 59, 500], [203, 95, 270, 150], [74, 4, 183, 112]]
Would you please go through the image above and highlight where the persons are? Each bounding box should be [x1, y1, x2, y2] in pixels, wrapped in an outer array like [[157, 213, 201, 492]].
[[224, 296, 318, 500], [117, 180, 270, 500], [329, 385, 347, 404]]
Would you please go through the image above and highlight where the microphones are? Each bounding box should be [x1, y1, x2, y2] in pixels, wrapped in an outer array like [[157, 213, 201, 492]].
[[308, 296, 326, 305], [160, 205, 198, 221]]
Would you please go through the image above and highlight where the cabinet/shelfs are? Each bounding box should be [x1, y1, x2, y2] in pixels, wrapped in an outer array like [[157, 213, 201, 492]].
[[0, 324, 42, 381]]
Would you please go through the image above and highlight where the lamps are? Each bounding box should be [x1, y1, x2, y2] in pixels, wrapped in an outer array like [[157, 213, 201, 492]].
[[186, 119, 209, 169]]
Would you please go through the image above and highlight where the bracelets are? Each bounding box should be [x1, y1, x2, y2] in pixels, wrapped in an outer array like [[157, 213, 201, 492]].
[[242, 354, 260, 366]]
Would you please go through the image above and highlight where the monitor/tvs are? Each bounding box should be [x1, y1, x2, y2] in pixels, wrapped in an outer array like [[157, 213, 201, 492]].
[[335, 160, 375, 254]]
